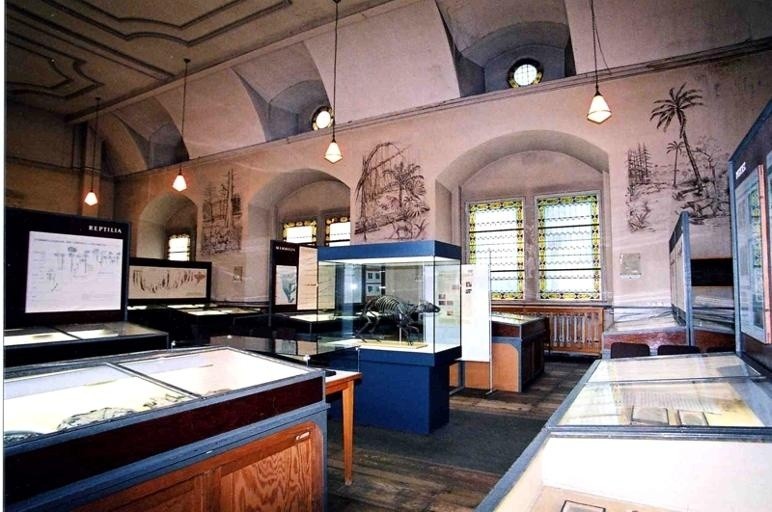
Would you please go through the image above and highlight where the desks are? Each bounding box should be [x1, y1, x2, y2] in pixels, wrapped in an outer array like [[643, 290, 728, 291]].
[[317, 367, 363, 487]]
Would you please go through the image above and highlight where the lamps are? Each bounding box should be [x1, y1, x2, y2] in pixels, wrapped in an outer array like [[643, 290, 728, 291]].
[[324, 0, 343, 164], [172, 58, 191, 192], [85, 97, 98, 206], [587, 0, 612, 124]]
